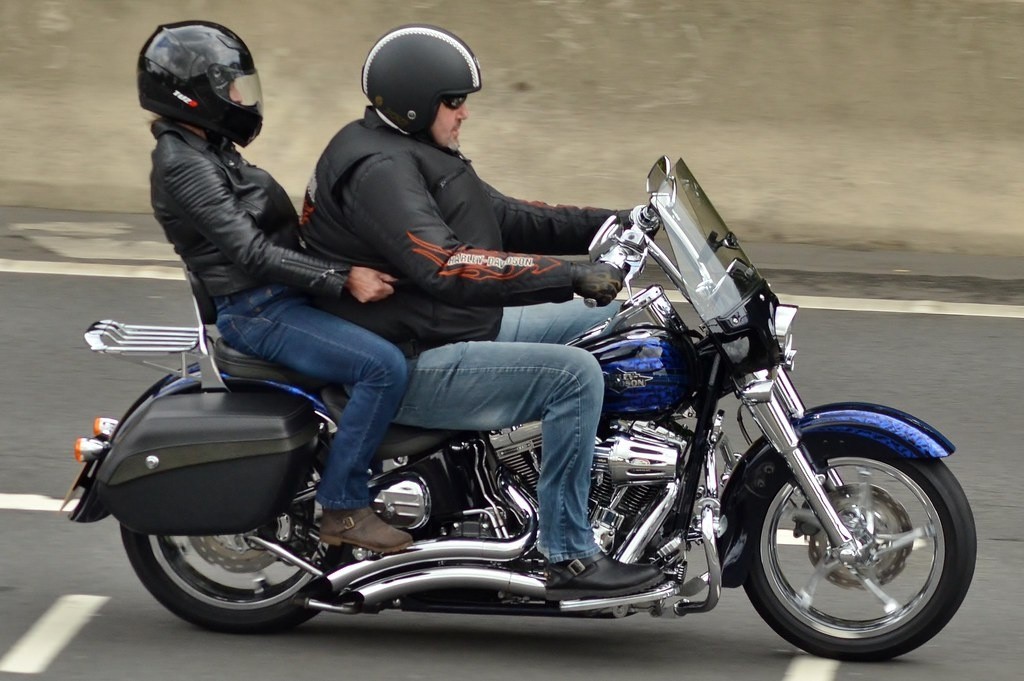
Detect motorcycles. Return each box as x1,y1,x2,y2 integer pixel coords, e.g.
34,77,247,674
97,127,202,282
59,155,977,663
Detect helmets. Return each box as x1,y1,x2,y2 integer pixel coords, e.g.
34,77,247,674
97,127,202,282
361,24,482,134
137,21,263,147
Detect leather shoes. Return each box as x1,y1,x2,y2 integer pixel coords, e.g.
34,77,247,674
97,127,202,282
544,551,664,601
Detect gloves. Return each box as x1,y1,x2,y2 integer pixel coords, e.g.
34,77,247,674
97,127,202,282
572,262,624,306
617,209,632,227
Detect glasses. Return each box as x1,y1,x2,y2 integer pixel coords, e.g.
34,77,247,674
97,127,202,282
442,95,466,109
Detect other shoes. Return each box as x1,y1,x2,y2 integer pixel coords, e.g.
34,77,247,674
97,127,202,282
320,508,414,551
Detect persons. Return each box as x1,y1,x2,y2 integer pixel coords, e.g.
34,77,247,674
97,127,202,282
138,18,414,554
301,25,668,602
